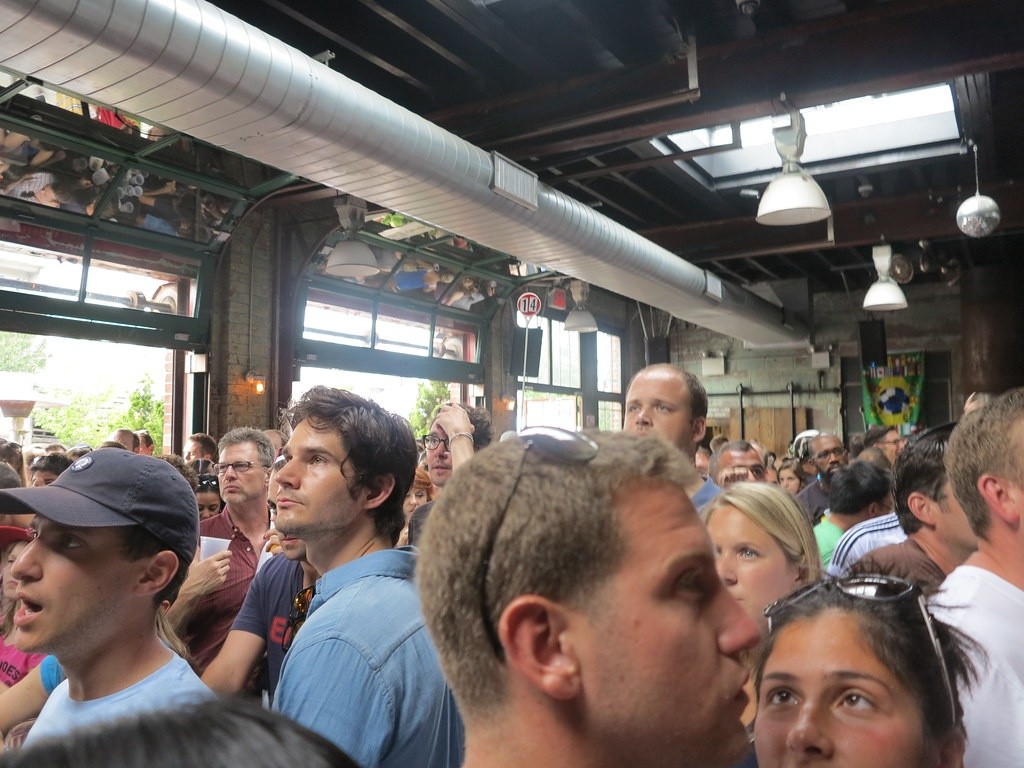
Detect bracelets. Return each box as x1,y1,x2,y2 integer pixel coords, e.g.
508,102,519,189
448,432,474,446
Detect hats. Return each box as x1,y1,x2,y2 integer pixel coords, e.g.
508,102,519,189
0,447,200,562
0,525,34,550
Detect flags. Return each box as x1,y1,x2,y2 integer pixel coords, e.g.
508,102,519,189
862,354,925,439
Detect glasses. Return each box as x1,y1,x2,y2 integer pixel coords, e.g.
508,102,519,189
212,461,271,475
197,478,219,490
281,584,315,652
879,437,906,447
422,434,451,453
478,425,601,663
763,573,958,725
32,455,63,465
811,448,849,462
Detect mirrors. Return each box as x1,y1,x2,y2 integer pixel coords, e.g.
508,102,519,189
475,258,549,278
0,71,22,90
382,256,460,303
426,236,501,263
0,83,172,156
441,272,506,316
144,138,286,191
309,231,406,289
358,211,450,249
0,128,121,217
98,168,235,244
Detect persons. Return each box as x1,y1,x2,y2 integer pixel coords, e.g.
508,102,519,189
339,213,497,313
1,383,465,768
416,359,1024,768
0,75,239,243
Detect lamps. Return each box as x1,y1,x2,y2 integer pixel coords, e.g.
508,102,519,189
245,369,266,394
754,107,831,227
324,196,380,278
562,279,598,334
502,392,516,412
699,349,728,377
862,244,909,312
807,342,835,369
